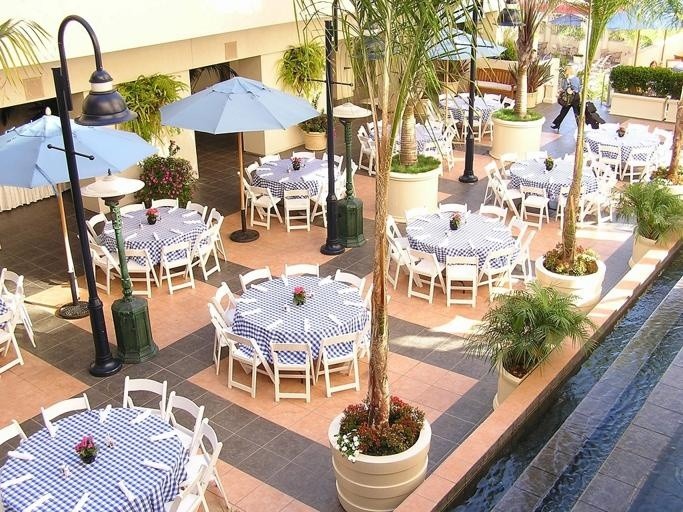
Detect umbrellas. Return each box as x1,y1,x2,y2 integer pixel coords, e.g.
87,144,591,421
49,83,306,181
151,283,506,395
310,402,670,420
425,25,508,126
643,6,683,63
605,9,664,67
0,106,159,306
549,14,588,26
159,76,321,231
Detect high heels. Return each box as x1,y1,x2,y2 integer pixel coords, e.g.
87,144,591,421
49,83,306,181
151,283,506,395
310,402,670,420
549,123,560,131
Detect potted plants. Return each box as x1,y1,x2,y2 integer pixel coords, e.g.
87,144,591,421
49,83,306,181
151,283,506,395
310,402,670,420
458,278,602,413
488,0,683,308
277,0,503,511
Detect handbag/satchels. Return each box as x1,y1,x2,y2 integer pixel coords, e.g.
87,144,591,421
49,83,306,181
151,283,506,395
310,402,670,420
557,90,575,107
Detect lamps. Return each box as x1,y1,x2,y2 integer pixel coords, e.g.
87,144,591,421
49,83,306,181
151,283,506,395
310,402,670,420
50,15,139,376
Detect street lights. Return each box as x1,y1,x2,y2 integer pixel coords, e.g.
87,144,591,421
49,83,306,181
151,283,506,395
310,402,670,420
458,0,524,183
44,15,125,380
319,0,386,255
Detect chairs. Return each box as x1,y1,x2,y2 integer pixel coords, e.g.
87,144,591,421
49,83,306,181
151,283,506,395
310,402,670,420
77,198,226,298
0,376,230,511
0,268,36,373
207,264,316,402
237,153,283,230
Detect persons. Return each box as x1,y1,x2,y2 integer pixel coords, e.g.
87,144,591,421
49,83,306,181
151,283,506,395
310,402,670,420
550,65,582,131
650,61,657,68
